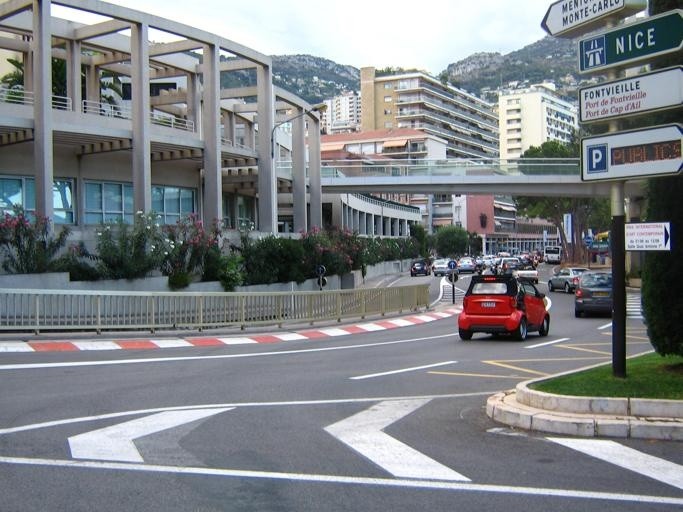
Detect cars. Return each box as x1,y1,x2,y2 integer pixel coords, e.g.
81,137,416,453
458,275,550,340
410,252,541,277
548,268,612,318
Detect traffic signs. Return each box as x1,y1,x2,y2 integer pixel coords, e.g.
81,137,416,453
540,0,647,37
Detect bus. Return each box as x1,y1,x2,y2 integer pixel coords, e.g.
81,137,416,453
543,246,563,263
